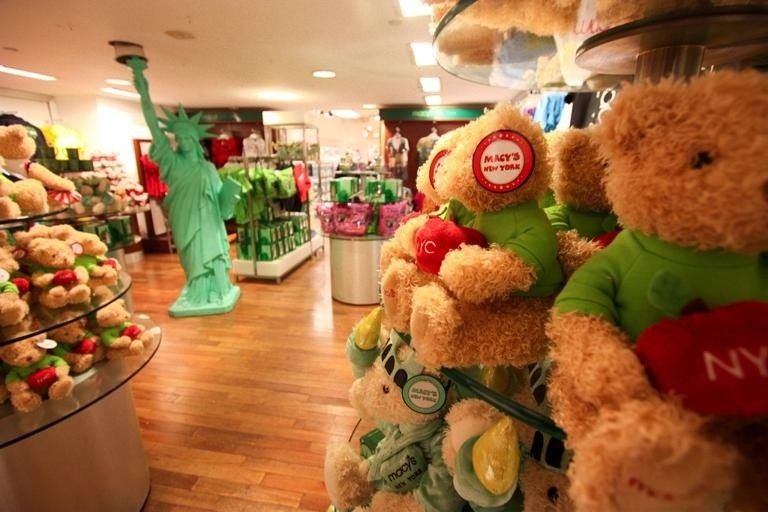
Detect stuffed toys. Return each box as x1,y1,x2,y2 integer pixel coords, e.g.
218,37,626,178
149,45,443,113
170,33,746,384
323,0,768,512
0,123,155,413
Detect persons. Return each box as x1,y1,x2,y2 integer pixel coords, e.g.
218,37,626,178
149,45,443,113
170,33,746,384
127,57,242,317
